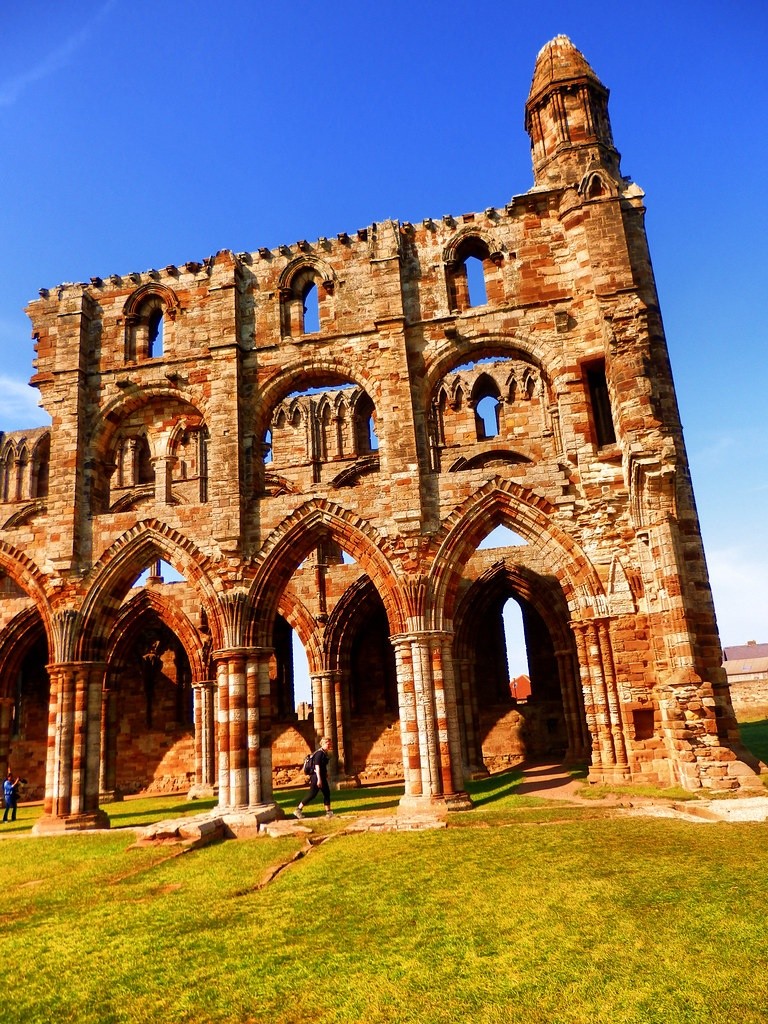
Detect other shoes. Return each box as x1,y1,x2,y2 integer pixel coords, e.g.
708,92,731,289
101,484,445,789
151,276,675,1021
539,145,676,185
293,809,305,819
326,810,336,818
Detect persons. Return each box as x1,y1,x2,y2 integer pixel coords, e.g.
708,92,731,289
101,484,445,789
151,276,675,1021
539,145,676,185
293,737,338,818
3,773,20,823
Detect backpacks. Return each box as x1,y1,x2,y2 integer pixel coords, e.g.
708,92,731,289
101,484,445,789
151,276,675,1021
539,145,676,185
304,750,327,774
